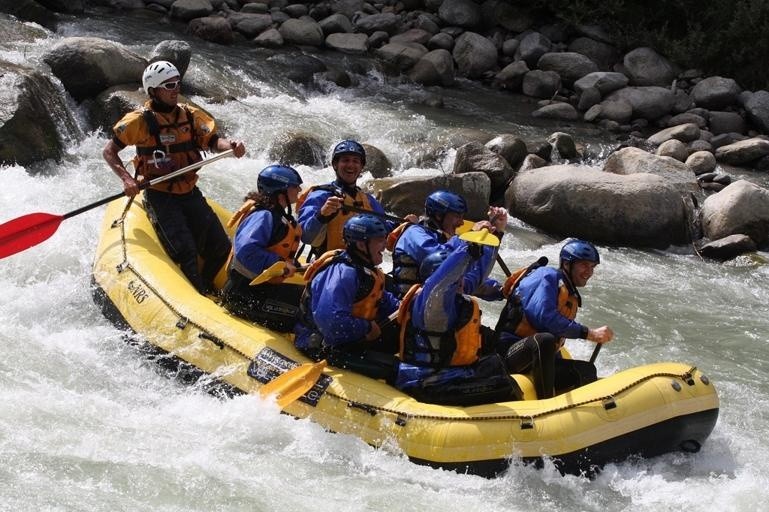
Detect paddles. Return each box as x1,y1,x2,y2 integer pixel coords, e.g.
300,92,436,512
338,202,474,237
0,146,236,260
248,260,311,286
459,207,502,250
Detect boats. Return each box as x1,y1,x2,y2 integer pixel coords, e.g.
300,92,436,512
88,189,720,483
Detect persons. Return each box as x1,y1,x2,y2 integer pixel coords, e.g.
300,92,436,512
397,206,524,407
392,190,512,301
294,213,400,390
220,165,312,334
495,240,613,400
295,141,418,262
103,60,246,297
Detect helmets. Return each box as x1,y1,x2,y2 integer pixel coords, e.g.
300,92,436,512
424,190,467,218
331,140,366,169
343,213,388,245
257,165,303,195
141,61,180,96
419,251,454,280
559,239,600,265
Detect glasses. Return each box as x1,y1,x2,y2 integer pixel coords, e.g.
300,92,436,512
158,79,181,90
